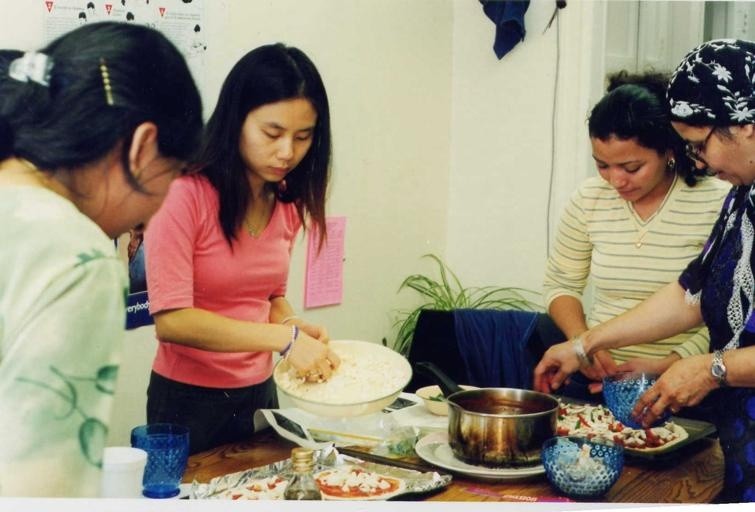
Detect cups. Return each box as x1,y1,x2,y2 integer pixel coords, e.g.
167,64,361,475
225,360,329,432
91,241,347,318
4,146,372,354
129,422,191,498
101,444,148,499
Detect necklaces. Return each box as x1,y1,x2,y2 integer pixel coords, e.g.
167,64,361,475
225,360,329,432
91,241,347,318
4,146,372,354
626,177,678,249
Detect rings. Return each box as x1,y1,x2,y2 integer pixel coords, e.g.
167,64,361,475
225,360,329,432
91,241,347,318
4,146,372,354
307,369,318,377
670,407,678,412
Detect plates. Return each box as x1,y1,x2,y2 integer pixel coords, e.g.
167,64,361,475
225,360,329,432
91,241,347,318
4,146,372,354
414,431,547,482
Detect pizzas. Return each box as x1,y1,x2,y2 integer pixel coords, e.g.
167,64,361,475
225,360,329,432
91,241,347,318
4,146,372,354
223,465,408,500
555,401,689,452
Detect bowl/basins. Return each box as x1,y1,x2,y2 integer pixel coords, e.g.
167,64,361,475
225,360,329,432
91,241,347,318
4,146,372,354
414,383,485,416
540,434,624,499
602,370,673,430
273,339,412,418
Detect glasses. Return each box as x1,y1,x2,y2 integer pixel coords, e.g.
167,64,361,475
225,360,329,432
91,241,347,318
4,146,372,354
683,126,718,162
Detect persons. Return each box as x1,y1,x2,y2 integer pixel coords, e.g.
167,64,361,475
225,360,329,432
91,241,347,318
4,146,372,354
533,37,754,501
1,23,203,499
542,64,733,395
146,42,339,457
127,232,147,292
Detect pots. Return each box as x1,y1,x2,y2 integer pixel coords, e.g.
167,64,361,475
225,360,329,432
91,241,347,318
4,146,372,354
413,358,560,468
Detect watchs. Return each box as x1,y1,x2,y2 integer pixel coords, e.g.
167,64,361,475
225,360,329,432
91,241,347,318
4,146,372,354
711,348,731,389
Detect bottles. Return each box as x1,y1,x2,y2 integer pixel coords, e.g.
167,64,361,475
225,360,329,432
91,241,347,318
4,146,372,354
281,446,323,501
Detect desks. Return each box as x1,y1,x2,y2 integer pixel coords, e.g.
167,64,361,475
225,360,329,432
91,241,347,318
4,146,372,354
180,398,726,503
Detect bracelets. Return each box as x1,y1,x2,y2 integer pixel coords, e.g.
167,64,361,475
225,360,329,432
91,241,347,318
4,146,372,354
573,335,593,368
282,316,300,325
280,325,299,355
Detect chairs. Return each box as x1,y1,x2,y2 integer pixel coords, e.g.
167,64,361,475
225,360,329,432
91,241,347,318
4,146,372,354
409,307,586,389
